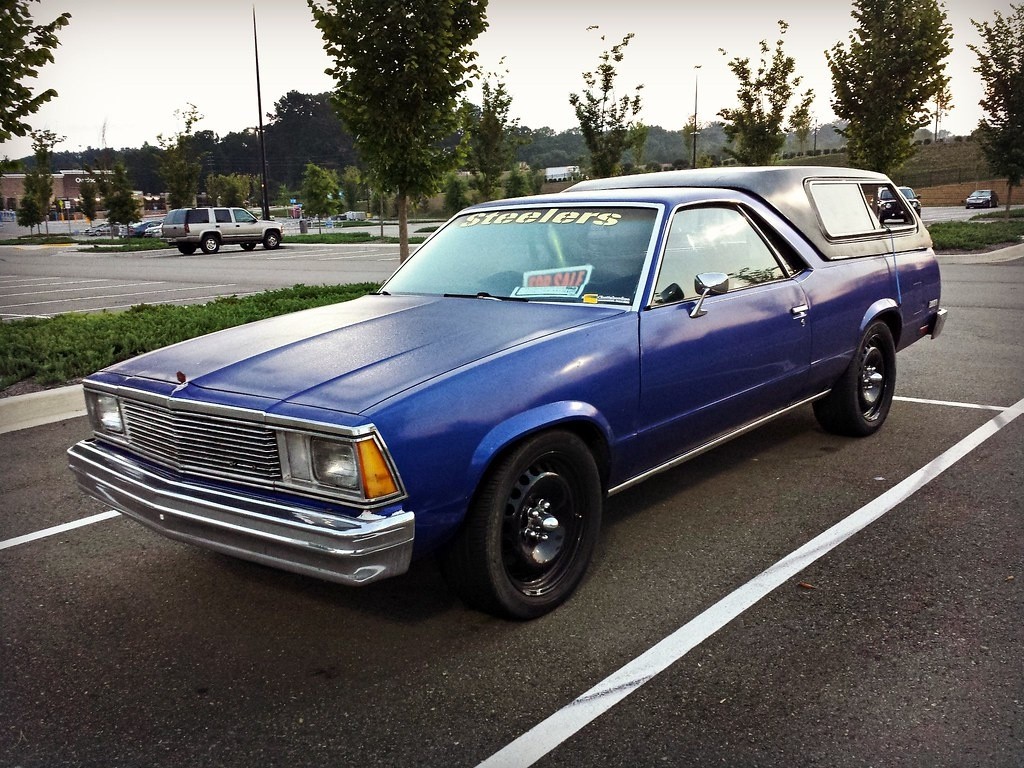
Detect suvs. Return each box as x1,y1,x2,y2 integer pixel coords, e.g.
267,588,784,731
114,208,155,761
162,206,286,255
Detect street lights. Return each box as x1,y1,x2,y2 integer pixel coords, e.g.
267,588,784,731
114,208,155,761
247,126,266,219
691,65,702,168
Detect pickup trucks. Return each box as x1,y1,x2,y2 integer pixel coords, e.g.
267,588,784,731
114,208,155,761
870,186,922,224
66,166,948,623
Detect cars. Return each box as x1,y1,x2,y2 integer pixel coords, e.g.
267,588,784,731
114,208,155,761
88,219,163,239
965,189,999,209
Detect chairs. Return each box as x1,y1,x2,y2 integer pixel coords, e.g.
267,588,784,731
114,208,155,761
325,221,335,233
